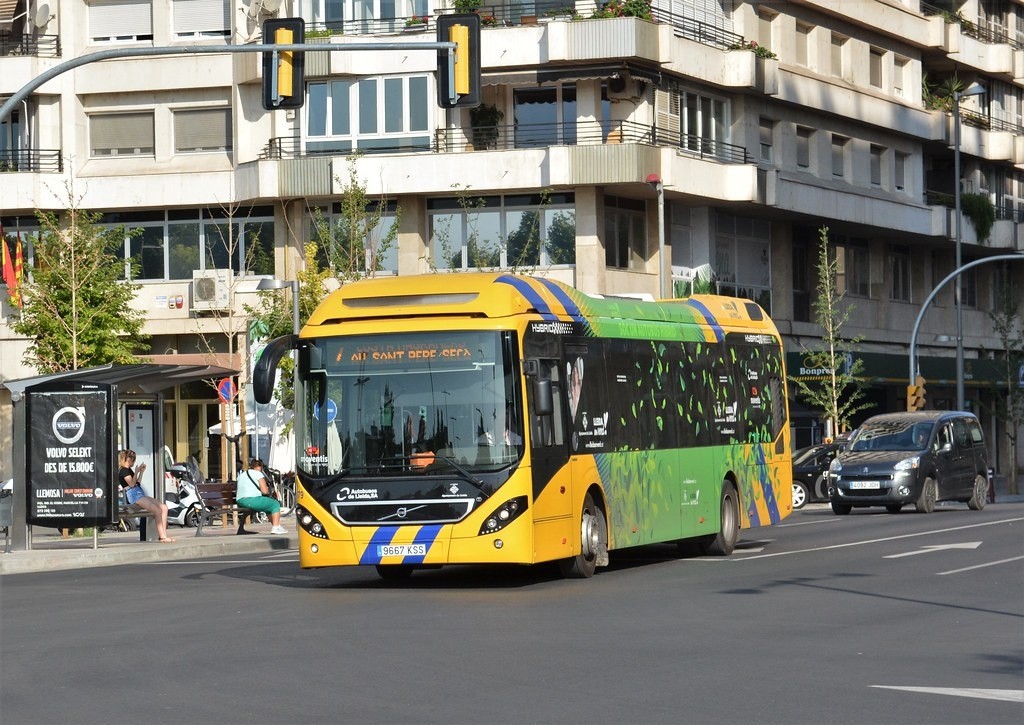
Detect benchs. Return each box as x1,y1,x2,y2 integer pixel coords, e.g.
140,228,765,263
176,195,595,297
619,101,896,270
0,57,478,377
119,510,155,542
195,483,255,540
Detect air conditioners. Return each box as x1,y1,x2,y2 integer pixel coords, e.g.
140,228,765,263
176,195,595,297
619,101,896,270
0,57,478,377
607,77,641,99
193,269,235,309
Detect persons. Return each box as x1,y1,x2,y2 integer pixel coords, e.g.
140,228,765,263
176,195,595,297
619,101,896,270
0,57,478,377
236,459,288,534
917,428,939,451
475,402,521,445
118,450,176,543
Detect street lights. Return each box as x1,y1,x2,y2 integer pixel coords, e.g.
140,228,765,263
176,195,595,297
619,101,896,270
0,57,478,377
255,278,300,372
950,78,987,412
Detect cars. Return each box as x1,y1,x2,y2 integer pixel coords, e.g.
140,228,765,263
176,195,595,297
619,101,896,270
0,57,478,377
790,442,852,509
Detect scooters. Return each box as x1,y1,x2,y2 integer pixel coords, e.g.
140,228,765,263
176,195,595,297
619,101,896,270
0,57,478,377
106,461,213,532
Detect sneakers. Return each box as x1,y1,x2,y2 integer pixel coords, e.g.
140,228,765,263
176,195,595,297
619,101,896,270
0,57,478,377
270,526,288,533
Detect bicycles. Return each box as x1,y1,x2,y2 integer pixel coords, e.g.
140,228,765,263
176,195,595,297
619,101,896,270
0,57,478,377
253,467,297,524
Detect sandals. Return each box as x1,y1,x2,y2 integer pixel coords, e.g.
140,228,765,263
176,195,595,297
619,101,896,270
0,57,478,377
159,537,177,543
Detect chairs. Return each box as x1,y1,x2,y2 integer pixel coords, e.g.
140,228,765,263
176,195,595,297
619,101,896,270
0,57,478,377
503,16,538,26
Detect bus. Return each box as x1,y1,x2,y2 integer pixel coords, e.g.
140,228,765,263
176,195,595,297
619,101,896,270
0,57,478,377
253,273,795,582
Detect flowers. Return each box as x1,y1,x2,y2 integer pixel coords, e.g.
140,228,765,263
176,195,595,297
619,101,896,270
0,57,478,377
405,15,428,24
484,15,496,24
598,0,655,22
744,41,761,51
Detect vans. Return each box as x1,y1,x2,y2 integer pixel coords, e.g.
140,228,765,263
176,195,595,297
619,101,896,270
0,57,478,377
827,410,991,514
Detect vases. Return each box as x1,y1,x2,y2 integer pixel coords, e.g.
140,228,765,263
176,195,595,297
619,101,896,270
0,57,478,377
402,25,426,32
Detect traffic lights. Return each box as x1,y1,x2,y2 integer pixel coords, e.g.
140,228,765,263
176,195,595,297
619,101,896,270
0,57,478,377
906,384,920,411
915,376,928,408
